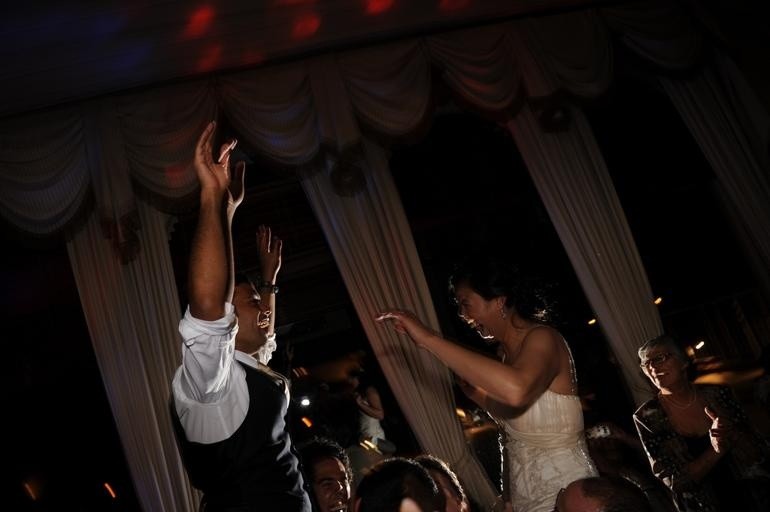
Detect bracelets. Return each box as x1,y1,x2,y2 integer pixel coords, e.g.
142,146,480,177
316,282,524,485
254,274,280,293
659,382,702,411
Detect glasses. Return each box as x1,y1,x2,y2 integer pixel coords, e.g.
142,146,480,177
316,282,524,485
639,351,677,368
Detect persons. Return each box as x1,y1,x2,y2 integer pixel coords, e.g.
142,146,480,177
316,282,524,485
632,333,769,511
168,119,314,512
370,241,602,512
554,476,654,511
352,457,446,511
415,454,467,511
345,365,387,442
294,436,353,512
